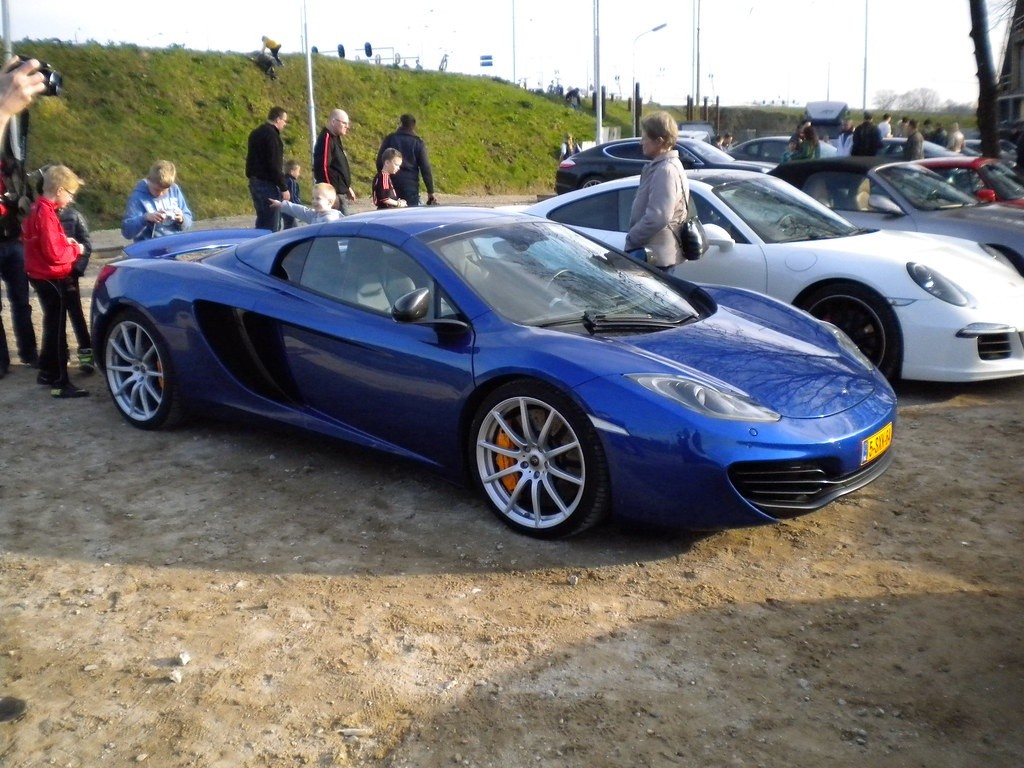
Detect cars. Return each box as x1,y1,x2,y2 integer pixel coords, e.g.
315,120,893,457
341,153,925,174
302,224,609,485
554,135,777,195
878,138,972,179
961,139,1021,157
725,140,839,163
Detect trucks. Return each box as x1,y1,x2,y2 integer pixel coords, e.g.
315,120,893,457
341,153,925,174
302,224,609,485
805,102,850,148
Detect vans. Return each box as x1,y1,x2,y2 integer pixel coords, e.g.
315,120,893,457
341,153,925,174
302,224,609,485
678,121,716,143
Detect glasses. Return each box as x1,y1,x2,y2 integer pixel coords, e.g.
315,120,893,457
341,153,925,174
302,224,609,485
65,189,78,199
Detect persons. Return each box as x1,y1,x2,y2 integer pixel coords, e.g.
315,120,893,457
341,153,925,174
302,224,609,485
0,36,439,397
712,112,1024,164
559,133,583,164
622,110,690,274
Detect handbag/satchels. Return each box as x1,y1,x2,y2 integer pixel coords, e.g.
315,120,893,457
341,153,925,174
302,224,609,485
680,216,709,261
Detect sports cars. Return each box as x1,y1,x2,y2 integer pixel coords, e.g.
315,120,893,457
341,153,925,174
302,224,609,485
736,156,1024,278
907,157,1023,206
470,170,1023,386
90,207,897,542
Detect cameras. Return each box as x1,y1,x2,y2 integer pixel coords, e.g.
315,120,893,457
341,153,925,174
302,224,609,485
8,54,62,96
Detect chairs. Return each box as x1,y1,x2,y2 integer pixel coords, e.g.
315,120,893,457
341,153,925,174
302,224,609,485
808,173,833,208
440,244,490,316
844,175,870,211
337,238,418,313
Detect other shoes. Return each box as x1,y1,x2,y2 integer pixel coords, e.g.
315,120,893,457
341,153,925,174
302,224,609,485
78,354,96,373
51,384,90,398
20,359,39,366
0,367,7,380
38,371,50,384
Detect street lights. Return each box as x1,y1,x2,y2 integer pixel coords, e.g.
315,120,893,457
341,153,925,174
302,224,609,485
632,23,669,138
